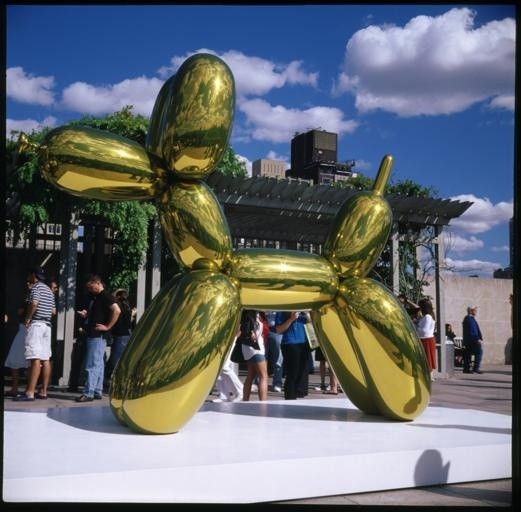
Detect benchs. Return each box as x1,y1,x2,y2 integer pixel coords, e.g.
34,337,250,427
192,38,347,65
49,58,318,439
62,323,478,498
453,338,474,366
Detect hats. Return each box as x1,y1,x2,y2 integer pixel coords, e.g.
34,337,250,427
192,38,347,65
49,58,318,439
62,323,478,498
113,288,126,297
468,304,478,310
31,267,47,281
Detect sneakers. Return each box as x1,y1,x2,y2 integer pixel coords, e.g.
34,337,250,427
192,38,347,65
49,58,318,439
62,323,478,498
93,394,104,400
75,395,93,402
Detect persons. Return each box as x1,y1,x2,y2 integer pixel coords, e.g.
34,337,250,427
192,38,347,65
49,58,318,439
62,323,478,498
461,306,484,374
104,287,132,392
241,309,269,404
314,345,333,391
402,299,436,382
14,271,56,401
211,337,246,404
5,283,57,401
35,281,59,391
323,360,338,395
72,282,114,392
445,325,463,368
398,296,421,320
276,308,312,400
265,310,286,393
76,276,122,402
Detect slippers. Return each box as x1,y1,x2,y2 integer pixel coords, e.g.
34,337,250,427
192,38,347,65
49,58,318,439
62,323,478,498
14,394,34,401
323,390,338,394
35,392,48,400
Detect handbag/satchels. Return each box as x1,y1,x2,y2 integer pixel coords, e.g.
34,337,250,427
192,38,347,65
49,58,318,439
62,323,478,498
303,322,321,349
231,345,248,364
